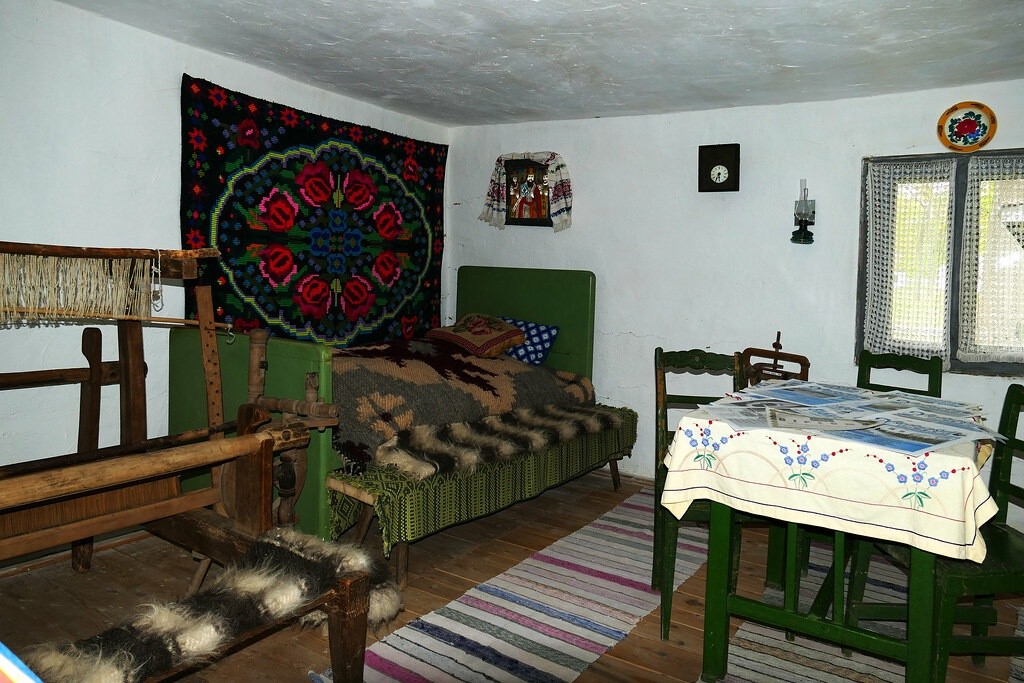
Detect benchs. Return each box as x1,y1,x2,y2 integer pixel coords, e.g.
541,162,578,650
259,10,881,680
21,574,336,683
326,402,637,592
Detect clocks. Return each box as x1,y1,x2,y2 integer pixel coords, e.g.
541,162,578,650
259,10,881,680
698,143,740,192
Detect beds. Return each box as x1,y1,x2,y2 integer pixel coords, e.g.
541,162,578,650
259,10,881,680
168,266,596,544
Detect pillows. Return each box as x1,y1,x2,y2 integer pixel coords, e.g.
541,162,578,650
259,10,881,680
426,312,525,358
497,316,560,367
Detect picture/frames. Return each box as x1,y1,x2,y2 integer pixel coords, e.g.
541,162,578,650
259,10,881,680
504,160,553,227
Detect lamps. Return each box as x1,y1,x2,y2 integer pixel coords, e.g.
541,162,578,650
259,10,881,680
790,177,816,245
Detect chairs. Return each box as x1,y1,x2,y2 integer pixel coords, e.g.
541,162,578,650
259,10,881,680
650,347,804,642
841,385,1024,683
799,348,943,592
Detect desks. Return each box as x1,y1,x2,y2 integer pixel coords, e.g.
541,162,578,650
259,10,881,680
628,379,1000,683
